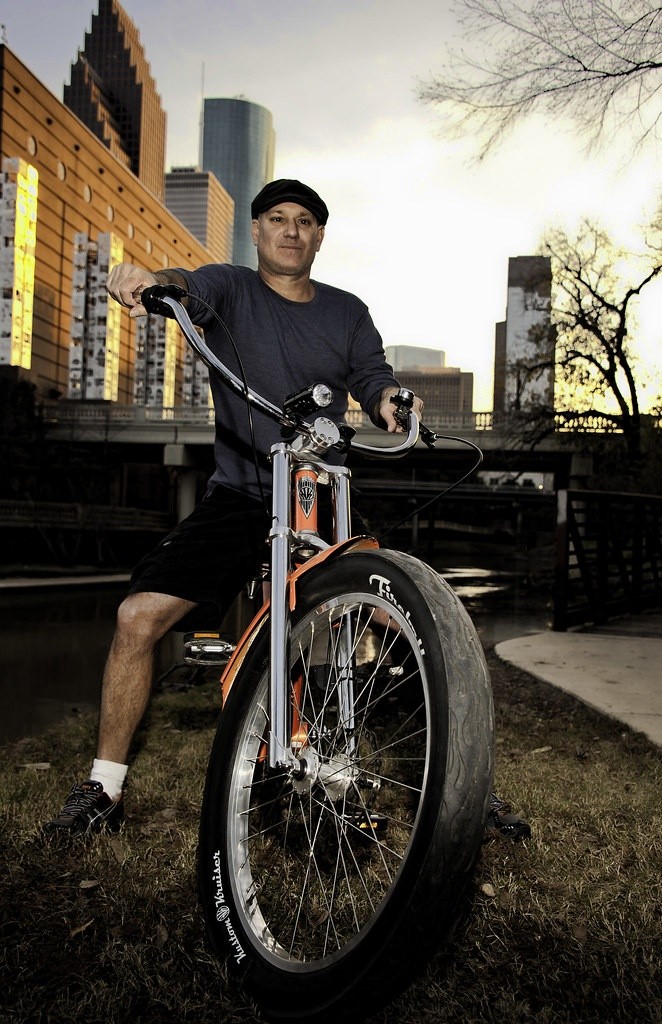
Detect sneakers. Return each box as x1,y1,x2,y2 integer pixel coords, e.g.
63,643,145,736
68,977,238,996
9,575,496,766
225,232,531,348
483,789,531,845
41,780,124,843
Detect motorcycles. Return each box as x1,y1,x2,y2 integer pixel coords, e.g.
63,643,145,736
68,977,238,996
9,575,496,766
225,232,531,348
140,285,498,1023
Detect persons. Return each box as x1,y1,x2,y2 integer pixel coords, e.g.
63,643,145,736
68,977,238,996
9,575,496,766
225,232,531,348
43,178,531,842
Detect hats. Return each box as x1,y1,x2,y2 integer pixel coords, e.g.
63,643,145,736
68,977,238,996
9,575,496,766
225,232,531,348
251,179,329,226
